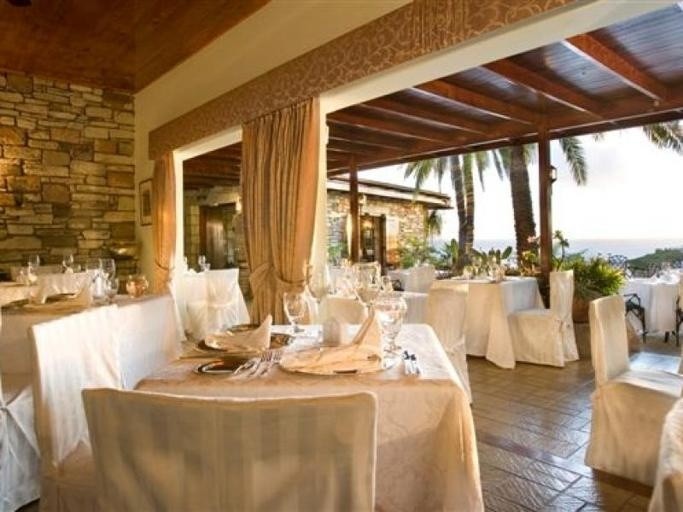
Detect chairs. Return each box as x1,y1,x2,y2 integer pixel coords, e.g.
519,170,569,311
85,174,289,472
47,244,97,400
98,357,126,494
419,287,474,404
81,246,253,336
27,300,119,512
80,384,379,511
585,295,681,485
300,247,682,368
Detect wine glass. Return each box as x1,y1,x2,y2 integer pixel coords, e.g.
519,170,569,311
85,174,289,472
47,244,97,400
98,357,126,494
472,256,497,279
86,258,119,305
282,258,408,353
197,255,206,272
29,253,40,278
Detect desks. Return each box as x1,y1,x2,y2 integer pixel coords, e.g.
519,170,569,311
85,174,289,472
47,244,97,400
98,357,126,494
134,325,484,512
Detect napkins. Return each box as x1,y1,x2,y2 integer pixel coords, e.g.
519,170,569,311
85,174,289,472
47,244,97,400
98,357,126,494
293,311,385,371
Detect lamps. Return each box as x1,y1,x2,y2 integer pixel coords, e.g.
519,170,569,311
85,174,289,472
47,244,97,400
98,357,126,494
360,212,373,231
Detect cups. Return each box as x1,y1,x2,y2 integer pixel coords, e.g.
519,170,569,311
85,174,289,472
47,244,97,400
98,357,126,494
202,262,210,272
126,274,149,301
62,252,75,268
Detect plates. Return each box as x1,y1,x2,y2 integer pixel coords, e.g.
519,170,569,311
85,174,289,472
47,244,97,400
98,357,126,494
196,323,296,375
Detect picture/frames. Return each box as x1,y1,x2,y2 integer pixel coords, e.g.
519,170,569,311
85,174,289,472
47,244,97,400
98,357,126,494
139,179,154,226
